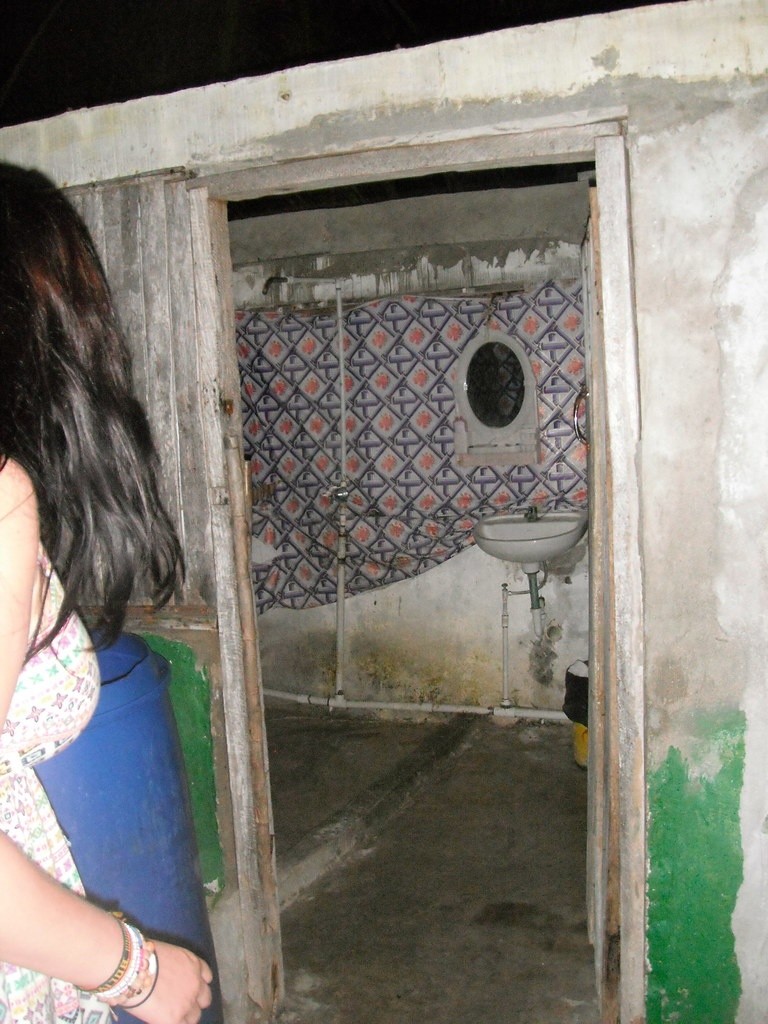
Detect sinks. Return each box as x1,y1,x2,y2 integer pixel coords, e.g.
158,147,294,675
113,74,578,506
472,511,590,563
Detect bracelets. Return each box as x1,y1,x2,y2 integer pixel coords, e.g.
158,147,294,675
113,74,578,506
76,912,159,1013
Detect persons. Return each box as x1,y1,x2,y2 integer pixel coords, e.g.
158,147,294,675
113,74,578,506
0,159,225,1022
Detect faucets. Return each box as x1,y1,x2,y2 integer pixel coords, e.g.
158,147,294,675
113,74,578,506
524,506,539,523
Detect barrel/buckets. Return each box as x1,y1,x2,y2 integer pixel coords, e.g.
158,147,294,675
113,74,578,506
34,630,223,1024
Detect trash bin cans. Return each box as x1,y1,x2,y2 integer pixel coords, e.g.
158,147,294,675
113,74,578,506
564,659,588,771
